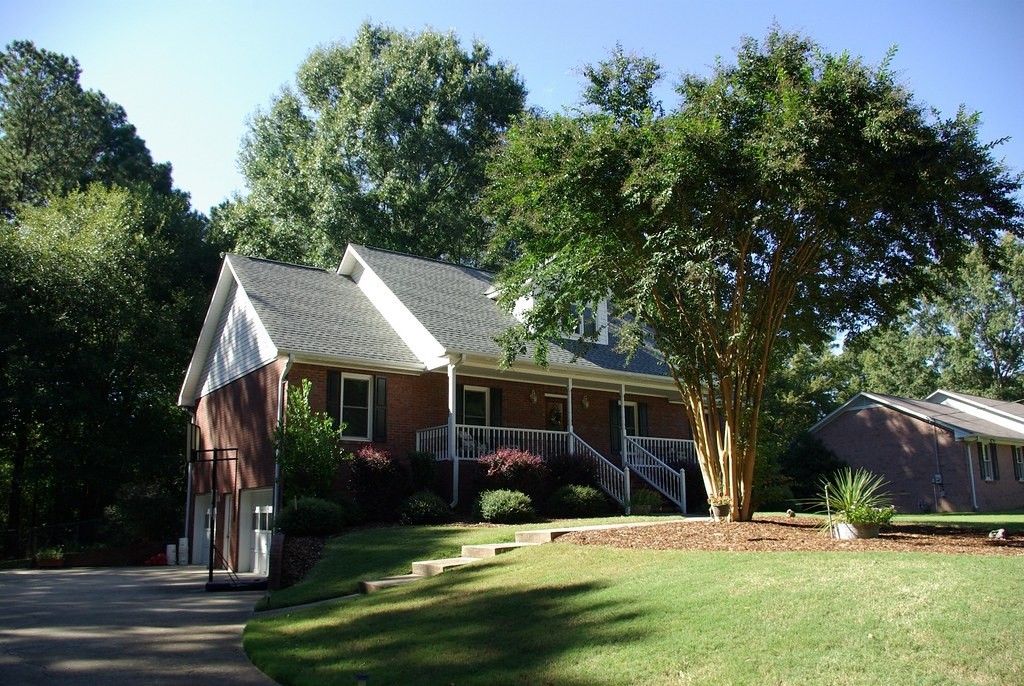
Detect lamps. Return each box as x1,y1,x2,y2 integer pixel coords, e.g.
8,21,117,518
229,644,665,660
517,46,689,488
529,389,538,405
582,395,589,409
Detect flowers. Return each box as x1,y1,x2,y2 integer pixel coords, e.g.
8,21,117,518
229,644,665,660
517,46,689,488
785,466,897,535
707,493,732,506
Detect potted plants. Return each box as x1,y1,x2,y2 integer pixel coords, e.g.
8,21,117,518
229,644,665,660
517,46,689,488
560,484,608,514
33,545,65,568
631,489,665,515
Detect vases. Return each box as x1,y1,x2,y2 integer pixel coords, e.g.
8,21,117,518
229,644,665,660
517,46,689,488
713,505,730,516
833,523,879,539
